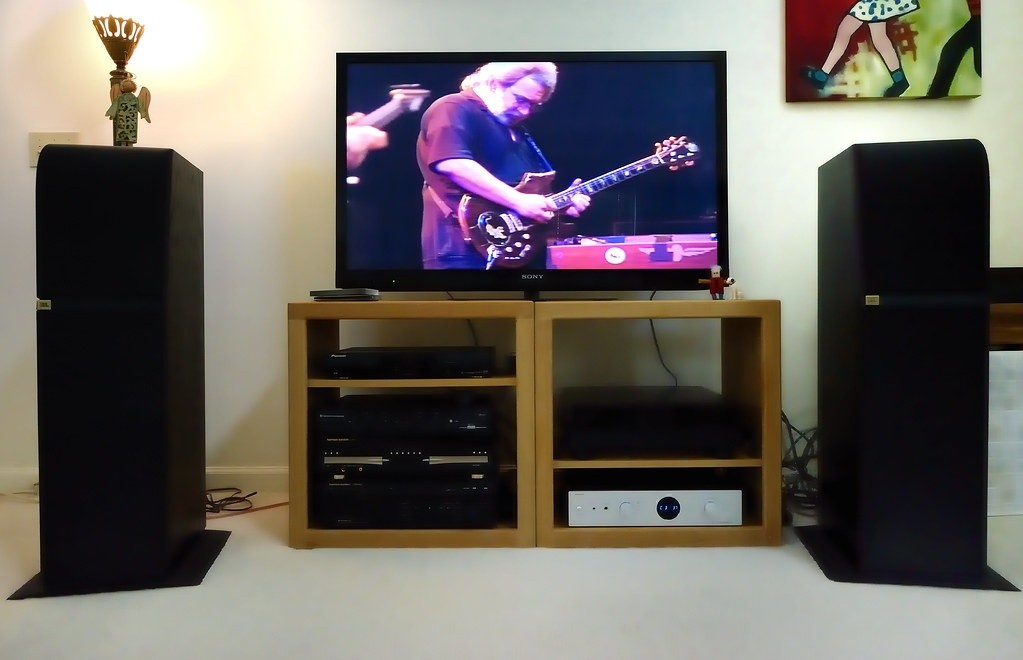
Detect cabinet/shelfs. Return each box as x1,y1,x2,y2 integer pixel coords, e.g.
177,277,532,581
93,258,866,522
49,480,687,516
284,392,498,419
284,288,785,549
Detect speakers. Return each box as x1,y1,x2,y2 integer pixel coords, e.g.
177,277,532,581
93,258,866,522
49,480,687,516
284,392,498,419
32,143,205,587
814,139,991,576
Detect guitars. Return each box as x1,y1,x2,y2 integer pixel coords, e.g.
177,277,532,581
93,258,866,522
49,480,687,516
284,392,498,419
456,137,699,269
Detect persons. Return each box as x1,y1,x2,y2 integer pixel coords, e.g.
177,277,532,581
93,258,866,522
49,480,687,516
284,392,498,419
345,110,389,173
416,61,590,271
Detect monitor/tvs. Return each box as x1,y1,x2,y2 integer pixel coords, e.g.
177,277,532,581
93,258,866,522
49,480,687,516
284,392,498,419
335,51,730,289
